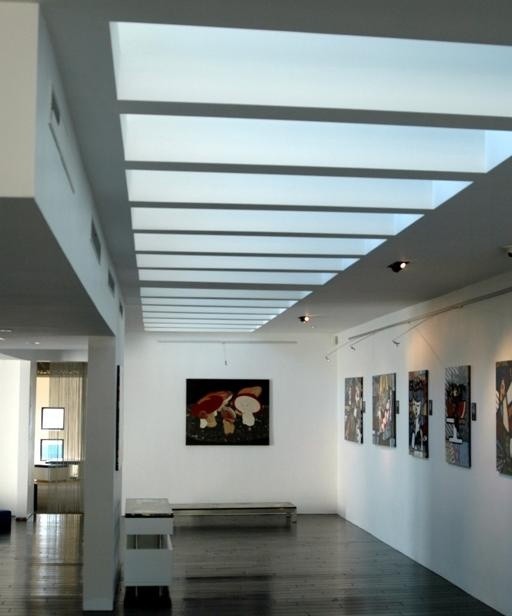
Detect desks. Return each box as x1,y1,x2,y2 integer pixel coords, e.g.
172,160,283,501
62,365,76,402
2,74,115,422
122,496,176,615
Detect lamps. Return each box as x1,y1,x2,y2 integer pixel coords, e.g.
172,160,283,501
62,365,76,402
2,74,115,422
388,259,409,273
298,316,310,322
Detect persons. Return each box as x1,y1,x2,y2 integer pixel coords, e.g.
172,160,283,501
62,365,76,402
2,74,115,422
409,378,427,452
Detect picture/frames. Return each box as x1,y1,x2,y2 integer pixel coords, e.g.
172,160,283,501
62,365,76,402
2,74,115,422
40,406,65,462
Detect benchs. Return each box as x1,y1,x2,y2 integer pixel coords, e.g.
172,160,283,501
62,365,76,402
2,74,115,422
171,501,298,528
34,464,72,483
